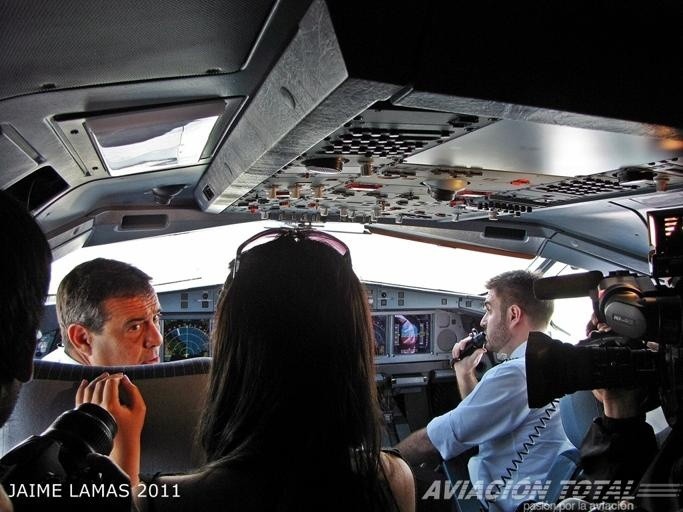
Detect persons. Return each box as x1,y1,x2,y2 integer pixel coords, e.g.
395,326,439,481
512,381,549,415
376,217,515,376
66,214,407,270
0,185,51,511
71,225,417,510
37,254,163,367
389,264,604,511
555,320,682,511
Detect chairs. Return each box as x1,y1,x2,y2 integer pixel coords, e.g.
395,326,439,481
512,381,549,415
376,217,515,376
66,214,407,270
0,355,221,511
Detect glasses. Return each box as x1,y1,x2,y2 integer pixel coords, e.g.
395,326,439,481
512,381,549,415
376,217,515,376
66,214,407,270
232,229,350,279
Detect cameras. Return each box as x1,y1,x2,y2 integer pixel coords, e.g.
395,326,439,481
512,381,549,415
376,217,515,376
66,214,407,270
0,402,118,512
